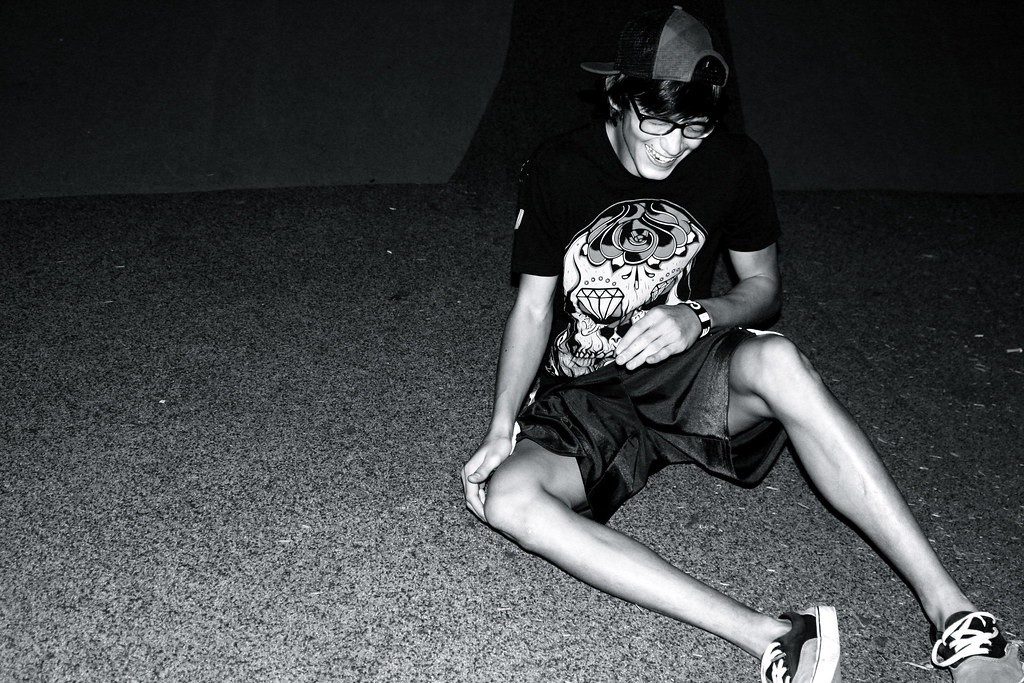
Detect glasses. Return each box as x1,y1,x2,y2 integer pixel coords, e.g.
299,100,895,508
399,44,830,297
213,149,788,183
626,91,717,139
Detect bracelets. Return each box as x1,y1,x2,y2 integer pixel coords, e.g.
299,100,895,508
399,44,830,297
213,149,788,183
677,301,711,338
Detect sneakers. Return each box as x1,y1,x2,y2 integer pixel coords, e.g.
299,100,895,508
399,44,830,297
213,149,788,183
931,610,1024,683
760,606,841,683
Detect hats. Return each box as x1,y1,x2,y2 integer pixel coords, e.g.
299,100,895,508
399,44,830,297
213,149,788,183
579,4,729,89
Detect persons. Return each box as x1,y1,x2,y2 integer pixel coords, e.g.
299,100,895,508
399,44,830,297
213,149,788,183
461,4,1024,683
